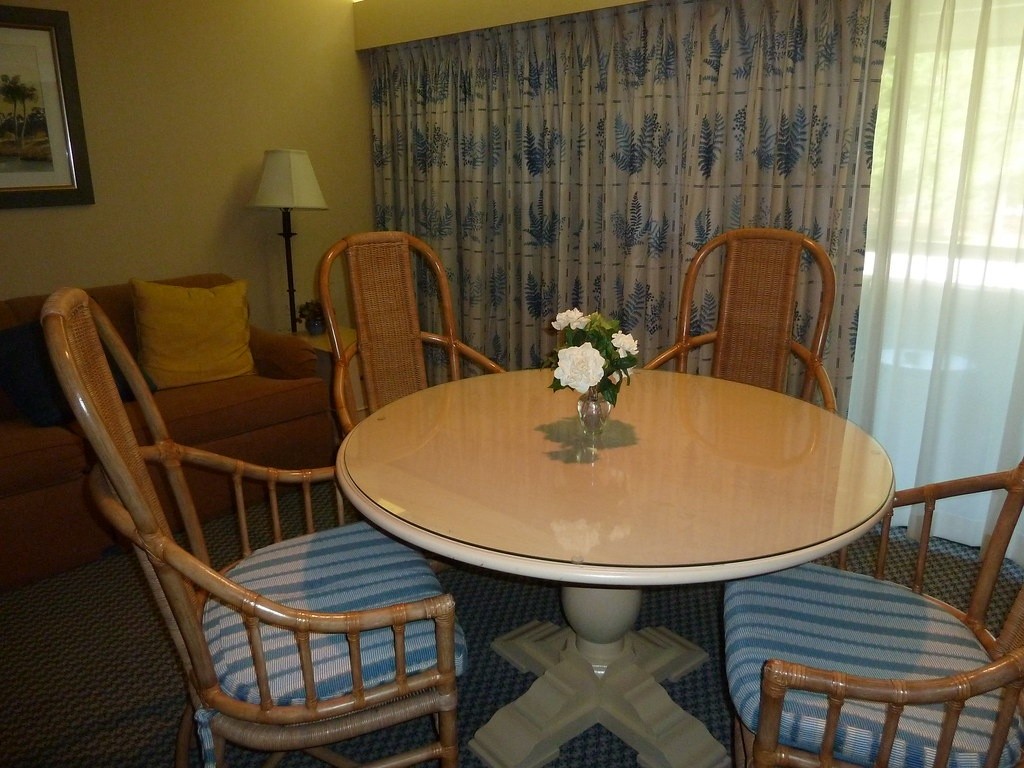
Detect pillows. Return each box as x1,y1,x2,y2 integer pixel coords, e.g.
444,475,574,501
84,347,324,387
127,278,255,391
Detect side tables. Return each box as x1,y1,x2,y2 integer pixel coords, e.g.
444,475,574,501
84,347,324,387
279,322,358,369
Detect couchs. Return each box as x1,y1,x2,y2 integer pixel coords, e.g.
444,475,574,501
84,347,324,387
0,272,333,586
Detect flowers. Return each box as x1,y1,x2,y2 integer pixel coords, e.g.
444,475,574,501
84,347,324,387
548,307,641,395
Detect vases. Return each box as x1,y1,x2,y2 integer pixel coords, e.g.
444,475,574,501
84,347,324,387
577,433,607,479
577,387,614,433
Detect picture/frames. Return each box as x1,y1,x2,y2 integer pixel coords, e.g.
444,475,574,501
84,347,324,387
0,4,97,209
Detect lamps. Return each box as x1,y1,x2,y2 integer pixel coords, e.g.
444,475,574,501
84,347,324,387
244,150,329,340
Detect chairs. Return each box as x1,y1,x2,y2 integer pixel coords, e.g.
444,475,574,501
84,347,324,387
315,229,511,434
39,285,467,765
634,229,840,415
726,447,1023,768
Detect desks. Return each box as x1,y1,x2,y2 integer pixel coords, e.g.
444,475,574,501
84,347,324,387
333,368,897,768
